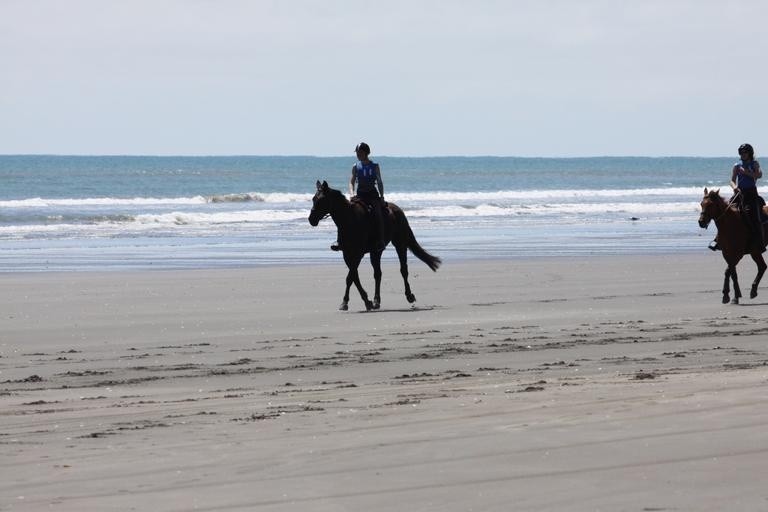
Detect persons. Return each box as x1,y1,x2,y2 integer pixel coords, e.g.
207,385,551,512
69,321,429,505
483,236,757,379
707,143,768,251
328,143,385,249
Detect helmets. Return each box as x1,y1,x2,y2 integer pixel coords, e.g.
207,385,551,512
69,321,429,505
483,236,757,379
355,143,370,154
738,143,753,155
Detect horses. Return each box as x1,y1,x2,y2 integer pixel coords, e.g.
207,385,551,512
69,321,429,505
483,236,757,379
697,187,768,304
307,179,442,311
